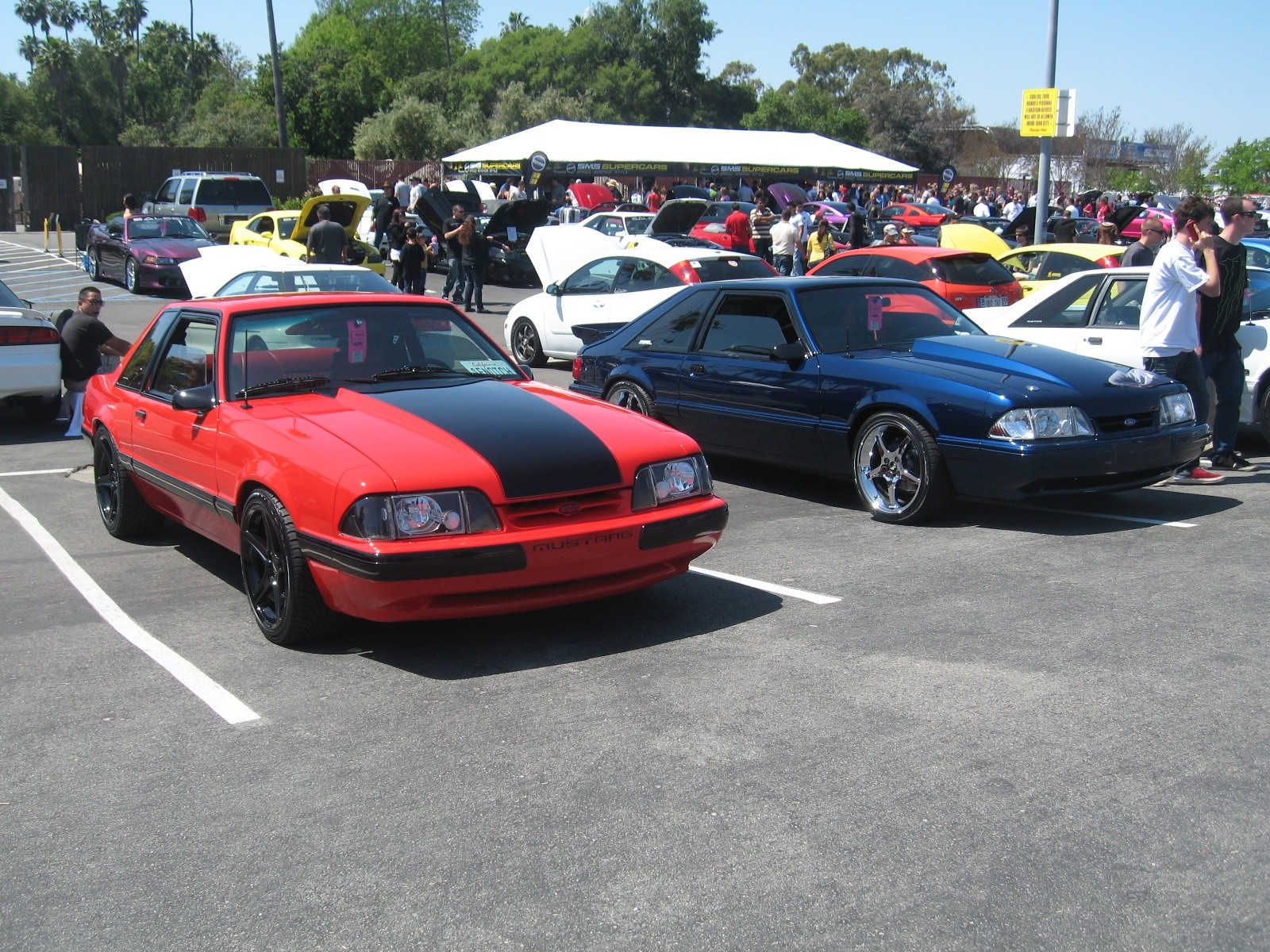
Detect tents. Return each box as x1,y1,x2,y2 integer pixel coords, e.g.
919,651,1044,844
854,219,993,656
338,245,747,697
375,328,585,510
439,119,920,203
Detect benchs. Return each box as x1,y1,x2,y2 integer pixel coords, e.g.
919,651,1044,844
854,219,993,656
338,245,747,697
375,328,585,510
161,345,337,396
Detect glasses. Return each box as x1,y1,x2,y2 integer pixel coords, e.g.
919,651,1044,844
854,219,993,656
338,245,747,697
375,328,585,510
455,210,464,213
1015,234,1024,237
1146,229,1163,235
81,298,105,307
1226,211,1257,218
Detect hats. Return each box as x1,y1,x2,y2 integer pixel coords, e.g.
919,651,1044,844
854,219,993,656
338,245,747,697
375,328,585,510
902,228,915,234
883,223,898,236
409,176,418,181
382,180,393,189
815,210,823,217
841,187,848,193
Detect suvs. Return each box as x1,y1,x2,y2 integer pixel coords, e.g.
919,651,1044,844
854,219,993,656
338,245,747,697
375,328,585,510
141,171,277,233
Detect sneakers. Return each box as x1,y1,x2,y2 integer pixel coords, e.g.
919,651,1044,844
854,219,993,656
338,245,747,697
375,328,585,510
1210,451,1261,473
1170,466,1225,486
1150,479,1169,488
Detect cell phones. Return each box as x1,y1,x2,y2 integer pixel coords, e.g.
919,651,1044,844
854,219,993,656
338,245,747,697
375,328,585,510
1185,220,1200,242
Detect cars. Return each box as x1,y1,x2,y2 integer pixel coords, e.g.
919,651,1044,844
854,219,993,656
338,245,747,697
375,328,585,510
318,180,1270,343
805,246,1025,328
81,291,730,648
568,273,1214,528
936,223,1129,307
952,263,1270,455
0,280,63,422
185,244,456,354
504,223,783,369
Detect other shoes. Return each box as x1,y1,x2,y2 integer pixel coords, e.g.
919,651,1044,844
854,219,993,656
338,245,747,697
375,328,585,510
453,297,465,304
464,307,476,312
442,295,448,301
476,309,492,314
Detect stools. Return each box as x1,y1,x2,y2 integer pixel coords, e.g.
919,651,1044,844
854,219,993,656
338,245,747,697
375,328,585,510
62,390,84,436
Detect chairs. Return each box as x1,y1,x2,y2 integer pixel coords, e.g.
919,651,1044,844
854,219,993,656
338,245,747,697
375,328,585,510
635,223,648,235
1106,305,1139,325
1252,251,1269,270
628,269,656,290
74,223,90,270
160,219,182,238
608,221,619,237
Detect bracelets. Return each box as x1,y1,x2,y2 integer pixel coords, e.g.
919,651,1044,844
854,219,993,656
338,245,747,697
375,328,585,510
305,255,310,258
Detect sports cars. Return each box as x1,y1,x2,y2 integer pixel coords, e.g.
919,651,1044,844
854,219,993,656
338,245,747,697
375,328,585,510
228,193,386,279
85,211,217,294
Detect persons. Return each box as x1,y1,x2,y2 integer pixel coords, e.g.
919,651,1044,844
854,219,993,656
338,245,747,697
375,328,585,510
1193,197,1258,471
124,193,141,218
490,178,527,200
429,235,439,254
725,198,835,276
305,206,350,264
704,182,762,204
551,178,582,202
1052,192,1140,245
1117,217,1163,295
60,287,132,392
808,183,1037,222
1016,224,1031,248
601,179,667,212
394,174,511,313
1139,196,1221,486
880,224,915,245
332,185,340,194
845,202,863,250
371,181,400,250
